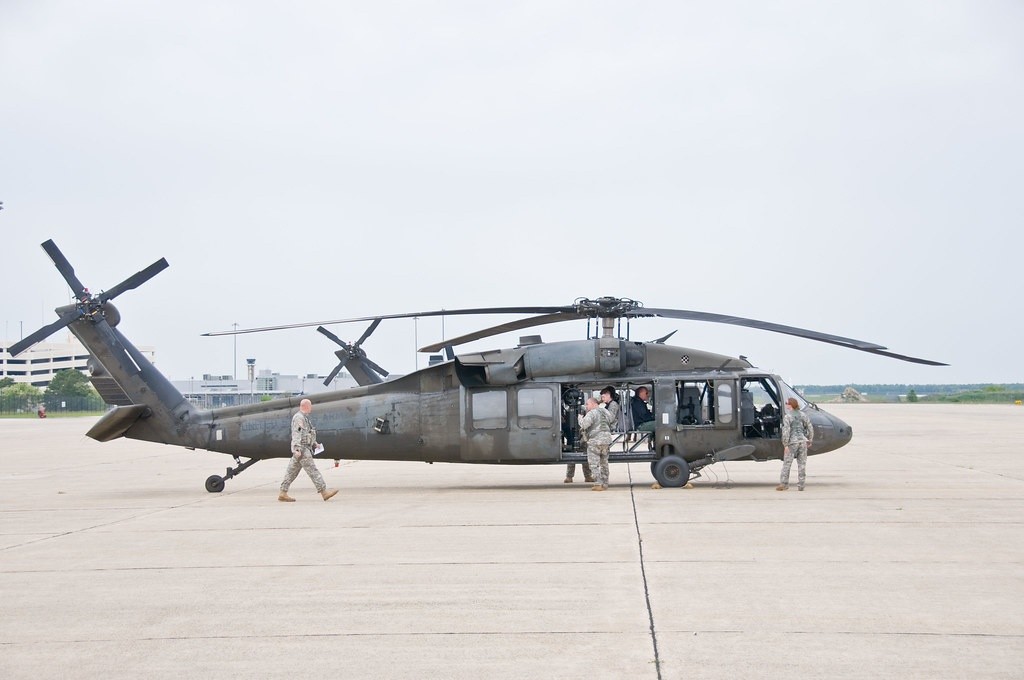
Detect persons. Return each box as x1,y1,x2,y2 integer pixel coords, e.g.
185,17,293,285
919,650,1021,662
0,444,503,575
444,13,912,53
776,397,814,491
560,463,594,482
594,386,622,432
631,386,654,431
577,399,614,491
278,399,339,503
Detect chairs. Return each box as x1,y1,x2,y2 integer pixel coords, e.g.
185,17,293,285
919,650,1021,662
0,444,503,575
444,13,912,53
607,389,626,453
624,390,654,452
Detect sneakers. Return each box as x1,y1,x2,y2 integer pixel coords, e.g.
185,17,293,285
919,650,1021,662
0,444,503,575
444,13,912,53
775,486,784,491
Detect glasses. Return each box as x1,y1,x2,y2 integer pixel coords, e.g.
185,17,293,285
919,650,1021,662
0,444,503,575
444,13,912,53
785,402,788,405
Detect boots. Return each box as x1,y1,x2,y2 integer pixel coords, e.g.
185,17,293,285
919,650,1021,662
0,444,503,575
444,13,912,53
592,485,604,491
320,489,339,501
278,490,296,502
564,477,573,483
585,477,595,482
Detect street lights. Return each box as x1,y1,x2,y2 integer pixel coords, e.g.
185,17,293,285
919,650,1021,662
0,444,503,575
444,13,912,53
231,323,240,380
412,317,419,372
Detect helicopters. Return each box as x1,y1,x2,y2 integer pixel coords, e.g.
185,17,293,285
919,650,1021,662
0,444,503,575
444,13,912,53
7,236,951,494
